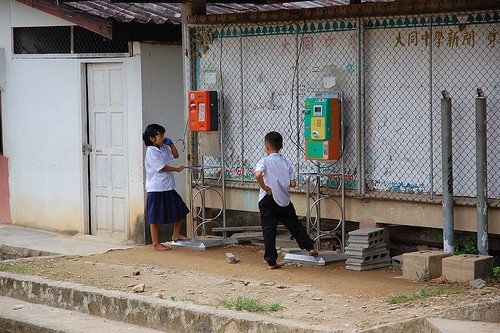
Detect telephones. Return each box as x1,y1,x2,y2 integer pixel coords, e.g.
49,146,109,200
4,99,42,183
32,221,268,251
164,138,171,145
303,97,339,161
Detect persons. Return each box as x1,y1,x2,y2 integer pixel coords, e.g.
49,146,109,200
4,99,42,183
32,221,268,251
255,131,318,269
142,124,192,251
